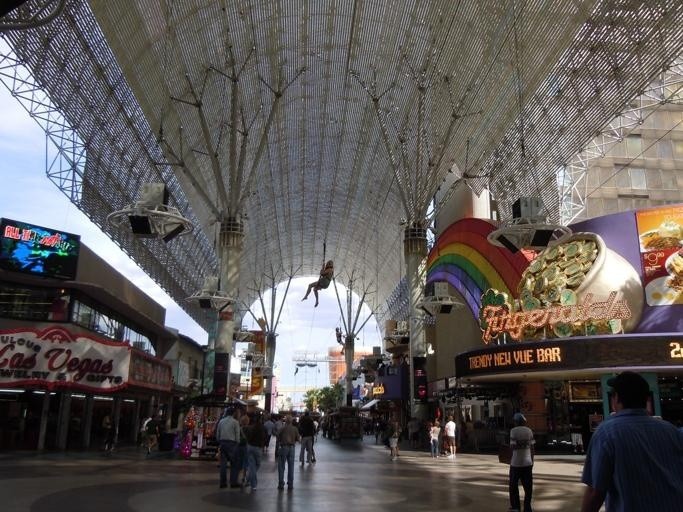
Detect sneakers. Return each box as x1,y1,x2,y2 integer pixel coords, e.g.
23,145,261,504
218,477,294,490
508,508,521,511
429,452,456,459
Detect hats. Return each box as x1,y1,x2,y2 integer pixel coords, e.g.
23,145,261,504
607,372,649,399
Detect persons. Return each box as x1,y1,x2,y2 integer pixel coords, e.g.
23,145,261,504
140,414,160,456
301,260,334,307
336,327,346,355
364,416,457,460
102,415,117,452
216,407,319,491
580,371,683,512
508,413,536,512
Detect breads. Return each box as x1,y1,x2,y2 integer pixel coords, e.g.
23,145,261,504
658,221,683,240
